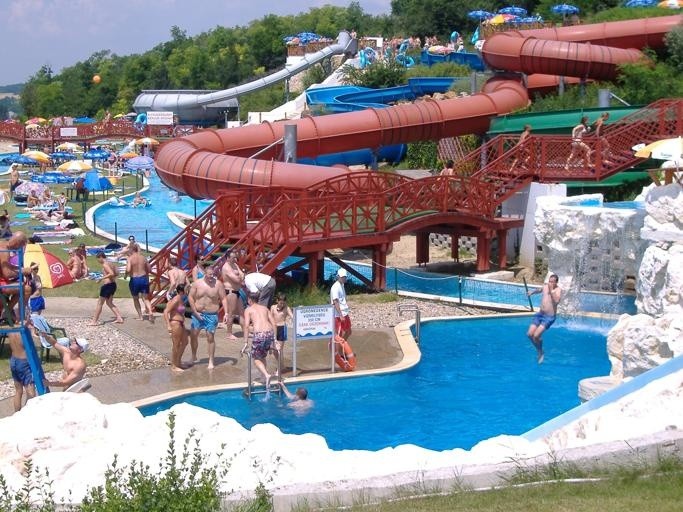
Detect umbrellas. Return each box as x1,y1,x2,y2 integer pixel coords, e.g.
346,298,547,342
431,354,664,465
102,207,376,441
282,31,332,47
632,135,683,188
463,1,682,37
0,109,160,186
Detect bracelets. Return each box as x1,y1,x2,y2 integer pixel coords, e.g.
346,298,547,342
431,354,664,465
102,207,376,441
550,290,554,294
243,341,247,343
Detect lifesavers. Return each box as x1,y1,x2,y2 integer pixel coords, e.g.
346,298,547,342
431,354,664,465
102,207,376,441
131,197,151,209
328,336,356,371
109,202,127,207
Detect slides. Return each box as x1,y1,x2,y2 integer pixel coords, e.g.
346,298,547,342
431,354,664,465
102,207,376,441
134,29,359,126
151,14,683,200
133,16,682,200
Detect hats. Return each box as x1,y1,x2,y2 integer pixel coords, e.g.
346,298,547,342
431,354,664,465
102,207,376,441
338,268,347,278
73,336,90,353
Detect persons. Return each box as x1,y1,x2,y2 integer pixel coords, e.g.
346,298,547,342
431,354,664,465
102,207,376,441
331,25,465,74
38,328,91,393
0,163,89,244
589,110,613,168
238,292,281,391
6,304,43,414
277,378,315,418
172,192,181,204
430,160,455,194
111,235,140,263
29,262,44,317
63,241,90,281
525,274,562,366
329,267,351,359
0,231,32,326
508,125,533,175
108,192,149,208
87,244,293,377
563,115,590,171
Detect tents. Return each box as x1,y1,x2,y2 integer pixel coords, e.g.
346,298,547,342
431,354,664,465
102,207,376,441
22,241,74,289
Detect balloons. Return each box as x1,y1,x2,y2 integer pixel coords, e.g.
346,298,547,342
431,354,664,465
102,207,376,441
92,75,101,84
